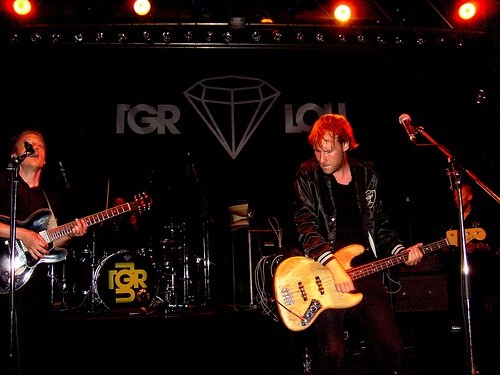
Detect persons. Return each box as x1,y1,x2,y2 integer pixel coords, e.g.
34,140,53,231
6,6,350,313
100,198,137,231
451,184,500,330
0,129,87,375
295,113,423,375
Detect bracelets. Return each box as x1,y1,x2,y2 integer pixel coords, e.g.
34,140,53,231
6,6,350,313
68,233,72,238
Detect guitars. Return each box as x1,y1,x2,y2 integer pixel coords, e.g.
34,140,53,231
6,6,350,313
272,220,487,336
0,192,154,294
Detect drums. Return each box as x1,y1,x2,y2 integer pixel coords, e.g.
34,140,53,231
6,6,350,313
159,222,187,246
24,249,68,309
93,251,159,313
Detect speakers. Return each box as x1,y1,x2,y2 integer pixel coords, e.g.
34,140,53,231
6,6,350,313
392,250,450,315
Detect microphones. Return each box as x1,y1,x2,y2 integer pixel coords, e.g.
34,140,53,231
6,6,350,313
23,141,37,157
399,114,417,145
185,152,190,177
58,162,70,188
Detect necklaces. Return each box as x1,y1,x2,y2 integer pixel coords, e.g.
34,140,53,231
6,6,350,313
113,214,121,231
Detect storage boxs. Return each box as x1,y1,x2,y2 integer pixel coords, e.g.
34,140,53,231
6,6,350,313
231,229,284,306
